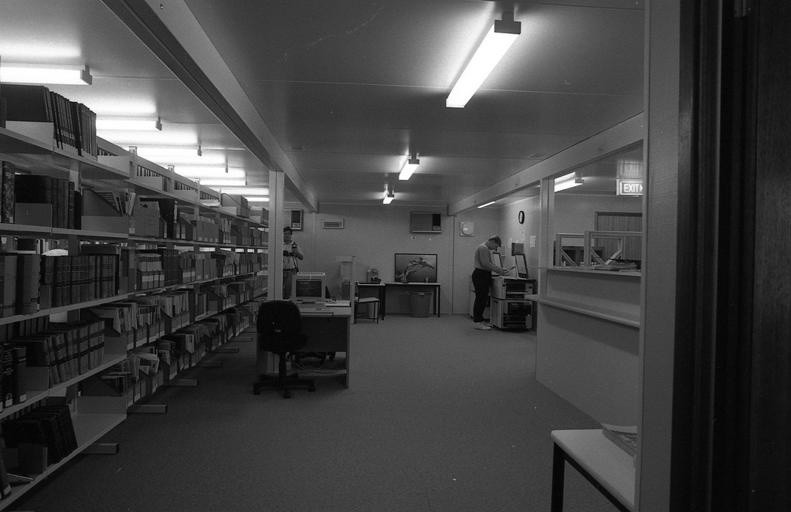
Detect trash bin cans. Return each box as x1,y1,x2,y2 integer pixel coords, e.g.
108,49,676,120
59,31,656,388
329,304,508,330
408,290,432,316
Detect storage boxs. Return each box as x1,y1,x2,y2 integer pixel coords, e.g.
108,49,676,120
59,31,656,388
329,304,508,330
249,206,270,224
221,193,248,218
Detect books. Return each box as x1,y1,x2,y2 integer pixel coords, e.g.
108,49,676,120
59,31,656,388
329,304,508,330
1,84,269,499
325,299,351,307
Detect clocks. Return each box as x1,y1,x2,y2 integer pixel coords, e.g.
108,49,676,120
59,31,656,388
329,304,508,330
518,211,526,223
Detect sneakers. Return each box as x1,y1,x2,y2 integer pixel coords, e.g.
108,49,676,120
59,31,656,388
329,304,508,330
472,321,491,330
482,320,491,326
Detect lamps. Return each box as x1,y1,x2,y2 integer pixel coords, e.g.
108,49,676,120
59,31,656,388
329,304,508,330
444,5,529,109
398,148,421,182
554,178,586,193
382,184,396,206
0,60,253,187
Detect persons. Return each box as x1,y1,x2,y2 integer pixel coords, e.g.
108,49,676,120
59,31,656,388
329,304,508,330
471,235,501,330
281,226,304,299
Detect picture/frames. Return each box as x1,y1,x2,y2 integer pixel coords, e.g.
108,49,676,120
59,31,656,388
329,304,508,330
394,253,438,284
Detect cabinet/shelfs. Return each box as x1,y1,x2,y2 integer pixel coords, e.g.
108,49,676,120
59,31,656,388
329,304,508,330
243,214,269,333
199,189,242,367
132,154,198,414
0,131,133,511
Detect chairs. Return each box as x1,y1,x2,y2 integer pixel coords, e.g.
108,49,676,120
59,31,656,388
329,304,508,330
348,280,380,323
254,299,311,397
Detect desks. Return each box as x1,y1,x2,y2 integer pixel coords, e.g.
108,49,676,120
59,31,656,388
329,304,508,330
257,296,353,388
383,283,443,320
550,425,639,511
256,271,328,298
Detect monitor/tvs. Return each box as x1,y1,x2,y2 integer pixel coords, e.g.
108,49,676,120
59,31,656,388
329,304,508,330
493,252,503,267
515,253,528,278
